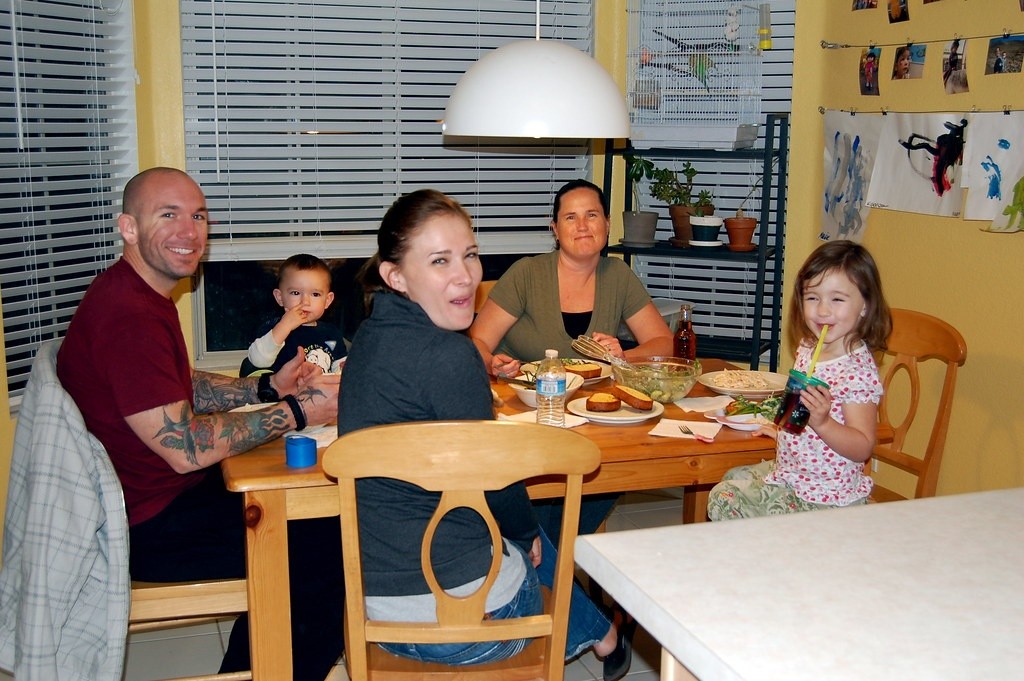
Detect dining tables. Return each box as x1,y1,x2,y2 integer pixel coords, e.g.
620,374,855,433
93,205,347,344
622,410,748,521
220,358,776,681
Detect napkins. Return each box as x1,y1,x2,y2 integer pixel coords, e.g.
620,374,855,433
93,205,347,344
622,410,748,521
497,410,590,429
648,417,724,440
672,395,736,413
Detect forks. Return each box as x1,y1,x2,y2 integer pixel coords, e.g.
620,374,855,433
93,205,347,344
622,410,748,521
678,425,714,443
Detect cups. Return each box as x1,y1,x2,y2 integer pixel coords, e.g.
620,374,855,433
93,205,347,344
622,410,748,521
285,433,317,469
775,371,831,436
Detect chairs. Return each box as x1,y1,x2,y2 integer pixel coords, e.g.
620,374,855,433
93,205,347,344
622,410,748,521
322,420,602,681
865,308,968,503
32,338,252,681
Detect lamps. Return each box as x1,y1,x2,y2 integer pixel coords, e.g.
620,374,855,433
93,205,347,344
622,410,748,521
441,0,632,139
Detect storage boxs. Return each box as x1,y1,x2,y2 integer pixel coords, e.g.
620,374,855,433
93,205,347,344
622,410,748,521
617,297,697,341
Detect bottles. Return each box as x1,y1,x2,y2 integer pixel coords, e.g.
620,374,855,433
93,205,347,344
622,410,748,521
536,348,566,429
671,304,696,372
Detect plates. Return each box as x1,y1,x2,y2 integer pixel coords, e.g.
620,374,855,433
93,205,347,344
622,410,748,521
567,395,665,424
698,370,790,399
229,402,322,437
519,358,614,386
702,408,765,432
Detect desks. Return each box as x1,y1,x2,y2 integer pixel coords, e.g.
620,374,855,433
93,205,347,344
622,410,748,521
573,486,1024,681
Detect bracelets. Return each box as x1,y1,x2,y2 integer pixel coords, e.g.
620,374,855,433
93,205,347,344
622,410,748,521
257,372,277,403
284,394,307,431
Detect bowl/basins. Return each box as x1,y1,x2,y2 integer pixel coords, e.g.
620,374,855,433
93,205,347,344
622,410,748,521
508,372,585,408
611,354,703,404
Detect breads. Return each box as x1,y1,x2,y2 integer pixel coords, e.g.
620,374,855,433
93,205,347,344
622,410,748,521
563,363,602,378
585,393,621,411
612,384,653,410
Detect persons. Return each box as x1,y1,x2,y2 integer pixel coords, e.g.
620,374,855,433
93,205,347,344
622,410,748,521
240,254,349,377
943,40,960,88
336,189,632,681
706,241,894,522
53,167,344,681
892,48,910,79
467,180,675,554
864,53,875,90
994,47,1006,73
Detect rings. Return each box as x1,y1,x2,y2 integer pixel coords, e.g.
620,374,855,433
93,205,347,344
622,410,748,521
607,345,611,351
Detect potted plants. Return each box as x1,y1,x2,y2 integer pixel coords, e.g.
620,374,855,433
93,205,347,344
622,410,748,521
619,155,779,250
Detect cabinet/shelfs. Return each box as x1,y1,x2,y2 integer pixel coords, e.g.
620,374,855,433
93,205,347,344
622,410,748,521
600,111,789,374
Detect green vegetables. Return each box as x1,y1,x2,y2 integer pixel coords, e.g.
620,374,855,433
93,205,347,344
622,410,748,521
617,358,700,400
726,393,781,420
560,358,574,364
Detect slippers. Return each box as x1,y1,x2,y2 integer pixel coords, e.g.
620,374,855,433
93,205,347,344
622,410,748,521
602,604,636,681
